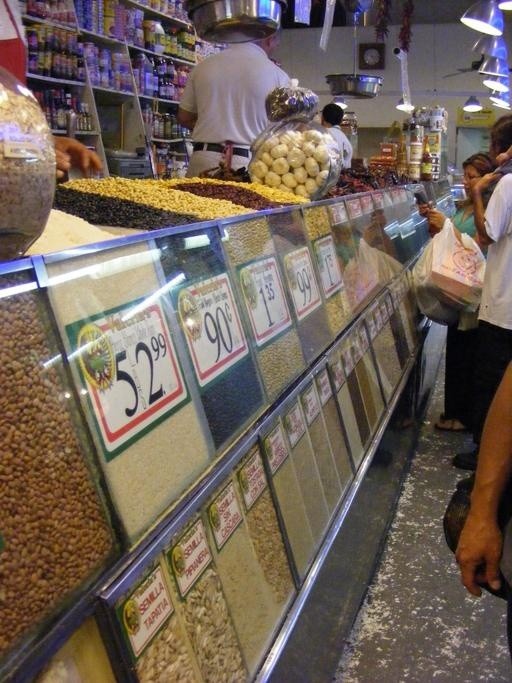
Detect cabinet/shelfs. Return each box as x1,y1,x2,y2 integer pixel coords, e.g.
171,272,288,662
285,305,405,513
1,175,452,683
0,0,231,178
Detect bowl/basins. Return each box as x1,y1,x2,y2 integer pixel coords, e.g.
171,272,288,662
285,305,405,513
326,74,384,99
187,1,288,44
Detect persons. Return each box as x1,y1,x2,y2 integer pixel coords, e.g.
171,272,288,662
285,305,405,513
177,30,291,178
418,153,494,427
322,103,353,169
453,115,512,471
0,0,104,178
495,146,512,166
455,359,512,664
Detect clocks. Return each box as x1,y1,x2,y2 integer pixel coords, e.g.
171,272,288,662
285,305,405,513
359,43,384,69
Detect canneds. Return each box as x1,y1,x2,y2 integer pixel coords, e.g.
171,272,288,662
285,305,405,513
410,142,422,162
408,162,421,183
167,26,195,62
26,23,85,83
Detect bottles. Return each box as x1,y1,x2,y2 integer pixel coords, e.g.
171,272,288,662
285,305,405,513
421,135,432,182
155,18,166,53
134,53,192,101
35,88,92,131
143,106,190,140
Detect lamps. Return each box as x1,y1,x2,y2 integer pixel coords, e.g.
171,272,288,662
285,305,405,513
472,32,509,61
499,0,511,12
484,75,510,94
477,58,510,77
491,104,511,112
489,95,510,105
329,98,347,110
463,96,483,112
460,1,505,38
395,97,415,112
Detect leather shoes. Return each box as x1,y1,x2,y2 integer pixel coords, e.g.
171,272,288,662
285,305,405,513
452,447,480,470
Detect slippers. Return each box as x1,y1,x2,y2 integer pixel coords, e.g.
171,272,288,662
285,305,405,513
435,418,466,431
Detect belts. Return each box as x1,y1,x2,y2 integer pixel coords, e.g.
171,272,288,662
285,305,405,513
193,141,249,157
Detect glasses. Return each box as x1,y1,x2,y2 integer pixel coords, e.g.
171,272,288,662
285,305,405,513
460,175,480,180
